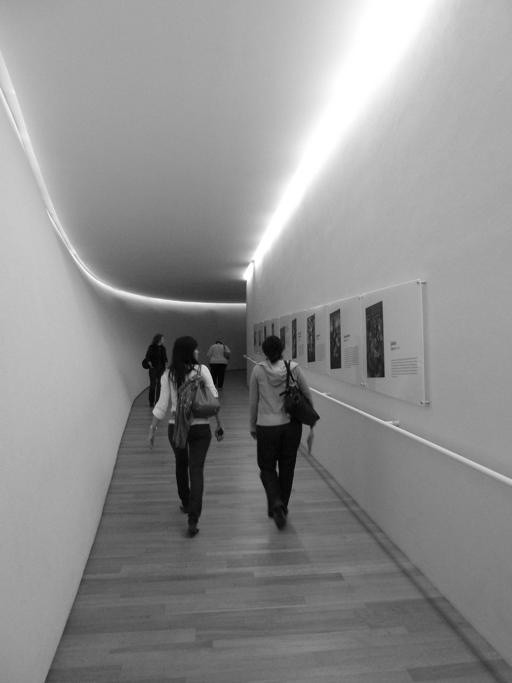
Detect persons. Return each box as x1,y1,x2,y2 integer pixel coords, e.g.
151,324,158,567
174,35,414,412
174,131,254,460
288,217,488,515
207,335,231,389
248,334,317,531
146,334,224,538
141,333,168,406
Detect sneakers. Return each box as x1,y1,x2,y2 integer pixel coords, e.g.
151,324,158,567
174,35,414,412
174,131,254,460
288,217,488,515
267,498,289,532
178,504,201,539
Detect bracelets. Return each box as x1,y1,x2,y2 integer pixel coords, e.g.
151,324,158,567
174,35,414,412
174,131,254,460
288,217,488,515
150,423,158,430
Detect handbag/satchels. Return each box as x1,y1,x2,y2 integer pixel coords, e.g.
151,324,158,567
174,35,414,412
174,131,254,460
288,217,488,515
184,371,221,420
280,383,320,426
142,359,149,370
224,351,231,360
